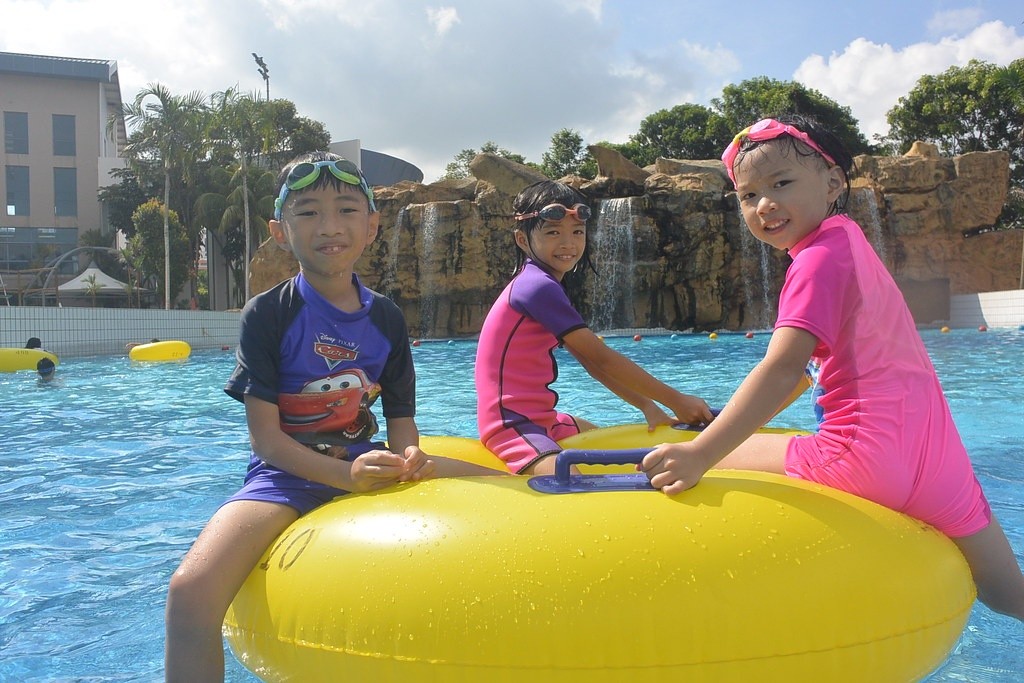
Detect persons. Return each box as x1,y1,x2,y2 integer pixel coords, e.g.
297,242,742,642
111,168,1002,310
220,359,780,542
125,335,159,351
159,141,438,680
24,337,48,355
470,174,715,488
633,108,1024,641
34,356,66,392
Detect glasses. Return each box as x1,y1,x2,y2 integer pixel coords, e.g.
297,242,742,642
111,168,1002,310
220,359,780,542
720,118,835,191
274,160,375,227
514,200,592,225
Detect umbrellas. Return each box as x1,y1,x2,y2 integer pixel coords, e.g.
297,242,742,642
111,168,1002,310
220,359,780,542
50,260,151,301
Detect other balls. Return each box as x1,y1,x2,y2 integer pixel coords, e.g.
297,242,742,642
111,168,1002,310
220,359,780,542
412,324,1024,347
222,345,229,350
